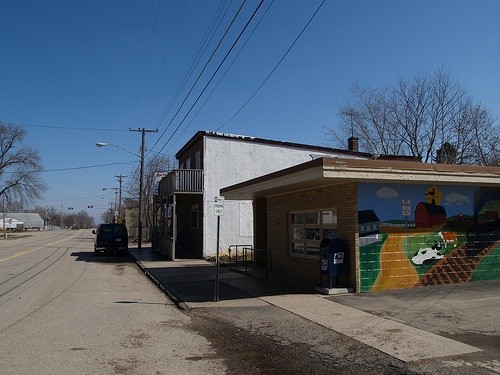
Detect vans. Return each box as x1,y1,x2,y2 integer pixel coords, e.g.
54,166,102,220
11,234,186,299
91,222,129,256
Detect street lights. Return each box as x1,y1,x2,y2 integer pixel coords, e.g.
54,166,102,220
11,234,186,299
94,128,145,249
101,187,119,221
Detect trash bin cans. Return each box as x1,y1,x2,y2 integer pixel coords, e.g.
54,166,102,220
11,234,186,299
318,237,351,290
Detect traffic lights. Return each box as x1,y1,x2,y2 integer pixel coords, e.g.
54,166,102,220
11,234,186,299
68,208,74,209
88,206,93,208
